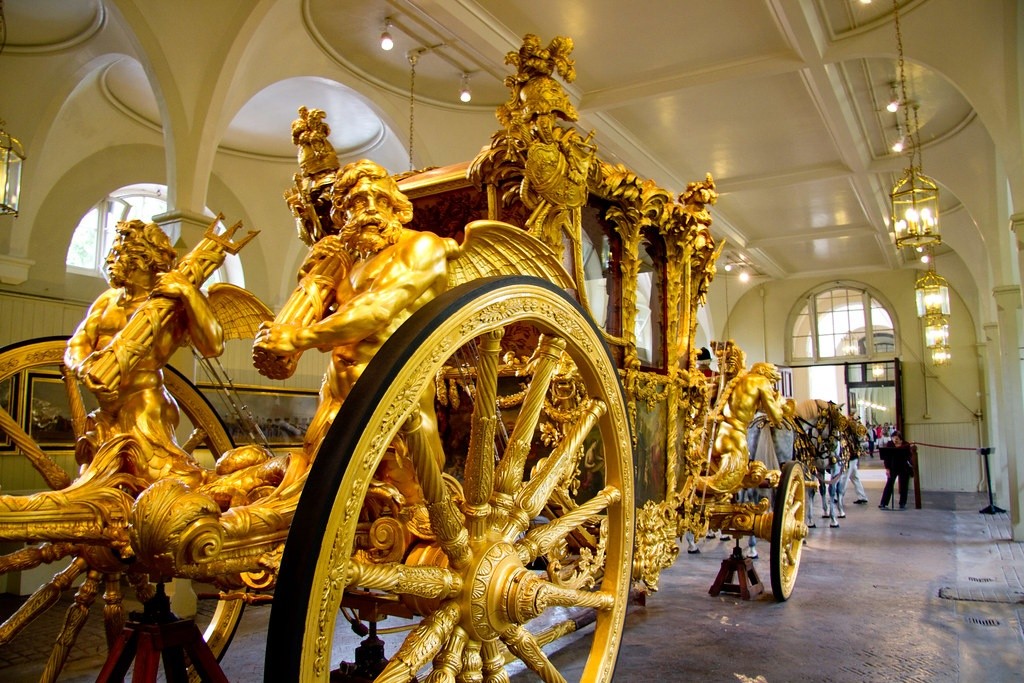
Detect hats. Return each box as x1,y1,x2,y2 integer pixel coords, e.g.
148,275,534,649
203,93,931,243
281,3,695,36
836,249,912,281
697,347,711,360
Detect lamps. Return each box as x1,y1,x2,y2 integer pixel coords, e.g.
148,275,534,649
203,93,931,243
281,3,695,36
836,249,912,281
378,17,396,51
840,331,859,357
915,268,950,368
740,264,749,281
886,93,901,113
725,256,734,272
0,119,28,220
871,364,885,379
459,71,473,103
889,167,942,248
890,135,906,154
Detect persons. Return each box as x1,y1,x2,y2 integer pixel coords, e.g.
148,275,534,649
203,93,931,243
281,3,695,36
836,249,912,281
696,347,720,379
865,422,897,458
258,160,447,507
831,438,868,503
717,360,788,467
878,430,914,508
64,221,223,477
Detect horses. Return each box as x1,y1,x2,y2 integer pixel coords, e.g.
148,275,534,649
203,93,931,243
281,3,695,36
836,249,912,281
685,400,869,559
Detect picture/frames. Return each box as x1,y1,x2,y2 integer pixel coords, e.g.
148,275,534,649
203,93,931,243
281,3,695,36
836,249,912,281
22,368,102,455
196,380,321,451
0,368,24,457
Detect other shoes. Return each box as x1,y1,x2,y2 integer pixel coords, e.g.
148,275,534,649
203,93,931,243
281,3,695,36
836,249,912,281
900,504,904,508
853,499,867,504
878,504,884,507
